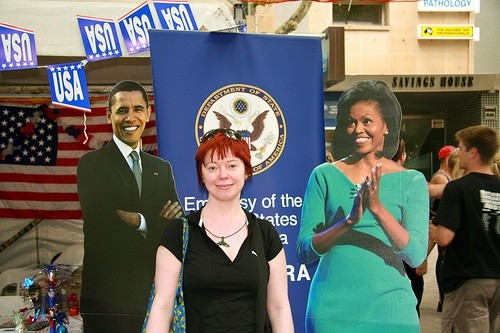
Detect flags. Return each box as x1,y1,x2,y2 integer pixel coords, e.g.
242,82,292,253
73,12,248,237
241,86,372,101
0,90,159,223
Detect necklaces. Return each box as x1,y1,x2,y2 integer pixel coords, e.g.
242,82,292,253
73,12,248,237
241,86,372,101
200,217,250,247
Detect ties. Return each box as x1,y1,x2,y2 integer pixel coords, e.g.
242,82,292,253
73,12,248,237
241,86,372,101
130,150,143,201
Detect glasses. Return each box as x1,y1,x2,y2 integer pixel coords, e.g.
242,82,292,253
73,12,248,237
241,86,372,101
200,128,242,142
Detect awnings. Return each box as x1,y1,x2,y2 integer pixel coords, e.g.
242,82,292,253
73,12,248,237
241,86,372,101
323,72,499,116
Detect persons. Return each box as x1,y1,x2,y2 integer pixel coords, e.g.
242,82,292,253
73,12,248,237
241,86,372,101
75,79,187,333
447,147,467,182
140,127,296,333
390,135,447,319
427,124,499,333
429,145,457,311
293,80,432,333
489,153,500,178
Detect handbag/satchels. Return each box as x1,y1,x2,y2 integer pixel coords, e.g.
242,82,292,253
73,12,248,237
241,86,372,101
141,217,188,333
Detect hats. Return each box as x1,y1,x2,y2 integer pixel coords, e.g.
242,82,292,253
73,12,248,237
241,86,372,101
438,145,456,159
